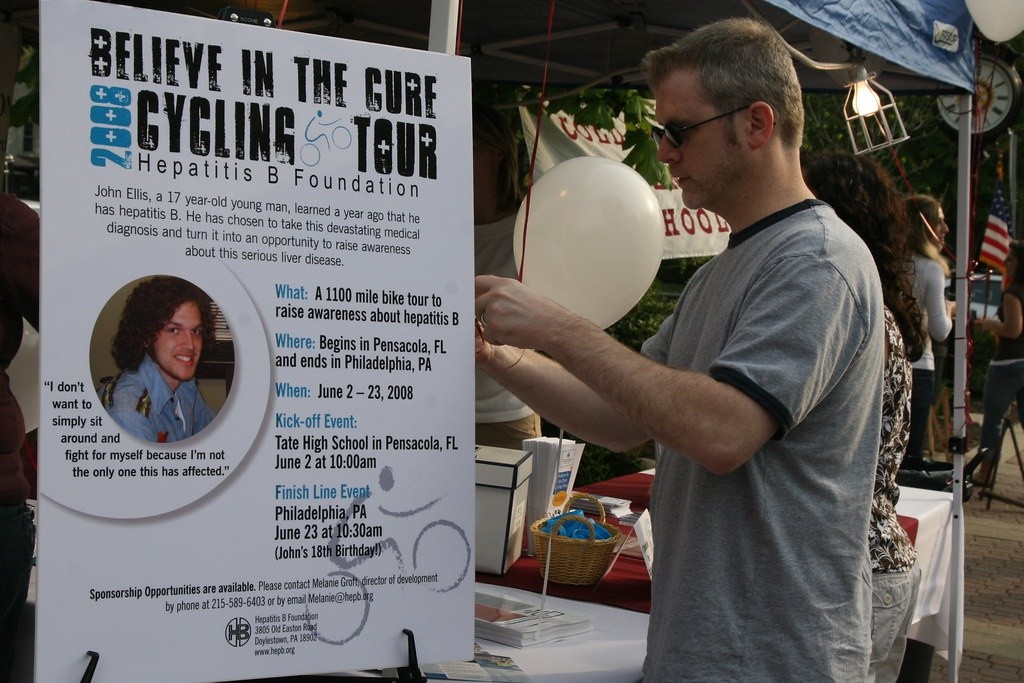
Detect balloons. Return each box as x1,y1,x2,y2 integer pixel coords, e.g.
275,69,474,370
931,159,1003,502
513,156,665,329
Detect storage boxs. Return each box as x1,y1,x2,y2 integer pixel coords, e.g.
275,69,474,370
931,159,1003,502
474,445,533,573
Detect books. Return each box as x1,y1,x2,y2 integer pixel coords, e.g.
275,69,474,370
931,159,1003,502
570,494,633,518
475,591,594,649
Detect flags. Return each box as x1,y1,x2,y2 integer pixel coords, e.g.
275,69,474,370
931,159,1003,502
981,186,1014,291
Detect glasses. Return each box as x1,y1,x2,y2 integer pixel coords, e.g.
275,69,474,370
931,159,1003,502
651,105,776,148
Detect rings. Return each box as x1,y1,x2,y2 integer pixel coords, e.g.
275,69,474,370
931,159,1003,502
480,314,488,325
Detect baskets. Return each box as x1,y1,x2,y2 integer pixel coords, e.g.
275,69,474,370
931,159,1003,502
528,493,621,584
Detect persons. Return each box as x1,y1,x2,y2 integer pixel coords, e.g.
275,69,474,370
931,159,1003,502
475,17,883,683
800,146,929,683
97,276,217,443
890,196,953,457
474,101,541,450
965,242,1024,487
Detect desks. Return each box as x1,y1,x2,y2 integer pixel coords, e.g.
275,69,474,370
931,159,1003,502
475,467,965,683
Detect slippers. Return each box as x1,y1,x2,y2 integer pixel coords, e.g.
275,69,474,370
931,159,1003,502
972,480,991,488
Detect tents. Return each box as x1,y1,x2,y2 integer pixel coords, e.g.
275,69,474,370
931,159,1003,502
424,0,977,683
